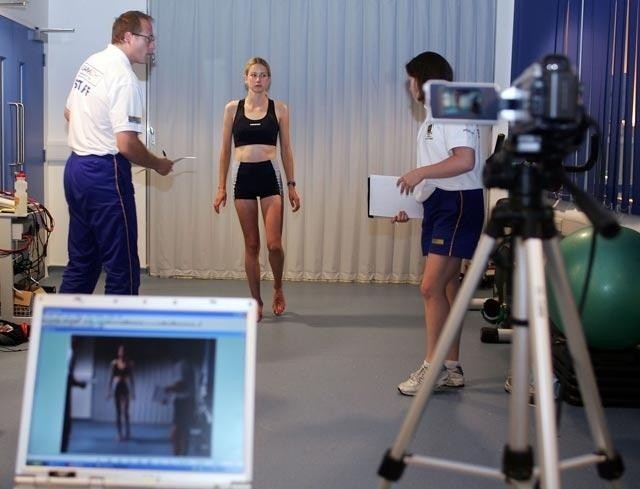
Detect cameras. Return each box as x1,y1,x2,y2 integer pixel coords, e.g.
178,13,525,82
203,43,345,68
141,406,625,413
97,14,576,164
423,51,586,133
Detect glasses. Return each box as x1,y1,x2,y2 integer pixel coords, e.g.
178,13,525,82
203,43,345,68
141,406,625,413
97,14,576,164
132,33,155,43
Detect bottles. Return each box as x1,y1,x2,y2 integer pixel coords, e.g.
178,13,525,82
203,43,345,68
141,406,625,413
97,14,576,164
14,173,28,216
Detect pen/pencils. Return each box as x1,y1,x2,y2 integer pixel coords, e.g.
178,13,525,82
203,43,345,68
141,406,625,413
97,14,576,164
163,151,167,158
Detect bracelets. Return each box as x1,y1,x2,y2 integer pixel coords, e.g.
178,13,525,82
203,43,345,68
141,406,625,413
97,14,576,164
287,181,296,186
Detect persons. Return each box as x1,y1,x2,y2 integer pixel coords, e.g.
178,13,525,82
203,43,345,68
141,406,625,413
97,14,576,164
390,51,485,396
214,57,300,324
57,349,86,452
154,347,197,456
106,345,137,443
58,10,173,295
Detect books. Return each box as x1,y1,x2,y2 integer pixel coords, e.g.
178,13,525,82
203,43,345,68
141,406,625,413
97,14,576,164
367,172,424,219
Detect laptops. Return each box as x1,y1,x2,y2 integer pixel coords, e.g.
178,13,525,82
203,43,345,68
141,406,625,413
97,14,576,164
11,293,260,489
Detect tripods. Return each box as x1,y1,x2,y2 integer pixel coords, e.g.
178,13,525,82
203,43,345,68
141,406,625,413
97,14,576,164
375,200,626,489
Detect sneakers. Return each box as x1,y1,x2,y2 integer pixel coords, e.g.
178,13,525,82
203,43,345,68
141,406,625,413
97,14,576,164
398,362,465,397
504,374,536,404
